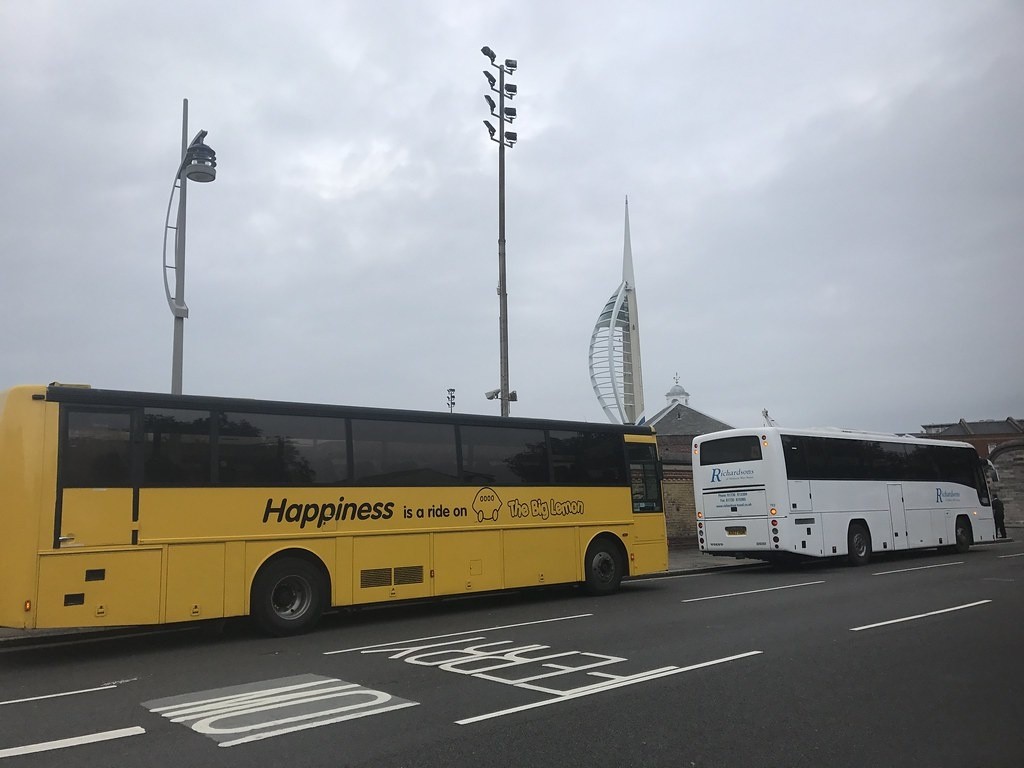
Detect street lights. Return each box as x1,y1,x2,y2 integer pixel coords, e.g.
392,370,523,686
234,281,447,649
481,45,518,417
162,98,217,438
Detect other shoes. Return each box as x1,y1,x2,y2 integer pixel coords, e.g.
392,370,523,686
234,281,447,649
1000,536,1006,538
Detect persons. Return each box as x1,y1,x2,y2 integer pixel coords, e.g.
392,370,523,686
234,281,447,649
992,494,1007,538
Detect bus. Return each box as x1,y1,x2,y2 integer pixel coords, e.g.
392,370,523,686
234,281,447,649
692,427,1002,567
0,382,669,638
